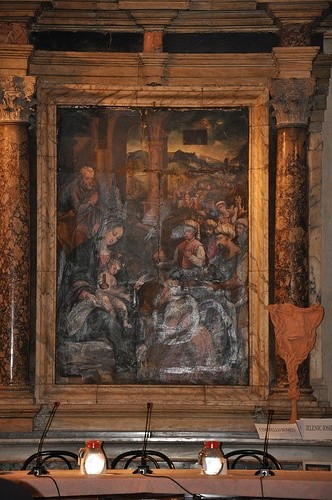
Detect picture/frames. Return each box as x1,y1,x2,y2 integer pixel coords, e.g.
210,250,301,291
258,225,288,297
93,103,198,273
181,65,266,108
33,84,268,403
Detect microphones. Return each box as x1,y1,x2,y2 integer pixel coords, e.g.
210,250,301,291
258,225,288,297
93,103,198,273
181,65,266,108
133,402,152,474
256,410,275,476
30,401,57,475
